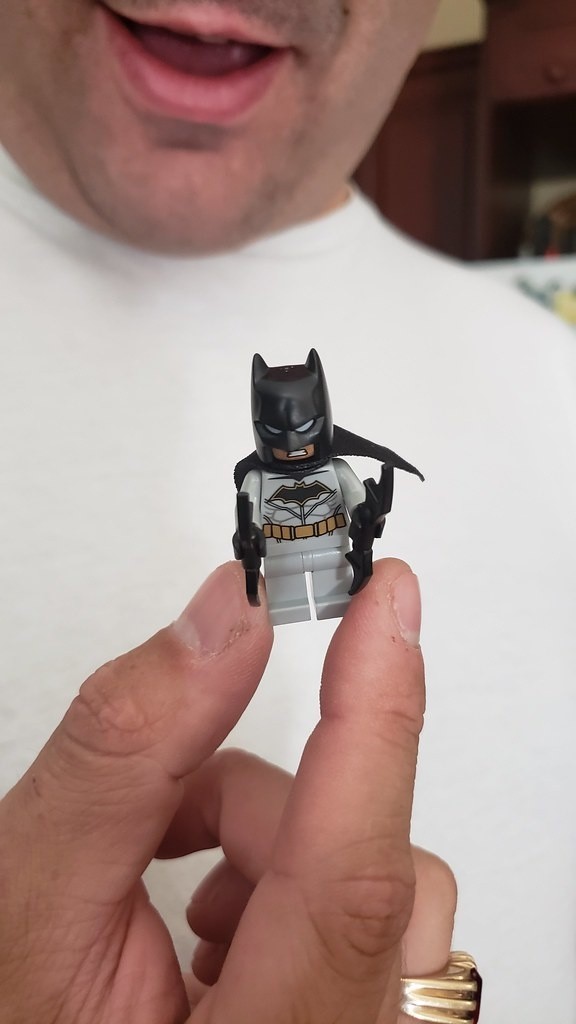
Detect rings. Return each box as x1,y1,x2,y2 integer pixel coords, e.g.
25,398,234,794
400,950,482,1024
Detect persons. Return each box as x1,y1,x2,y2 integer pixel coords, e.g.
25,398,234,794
231,348,425,626
0,0,576,1024
